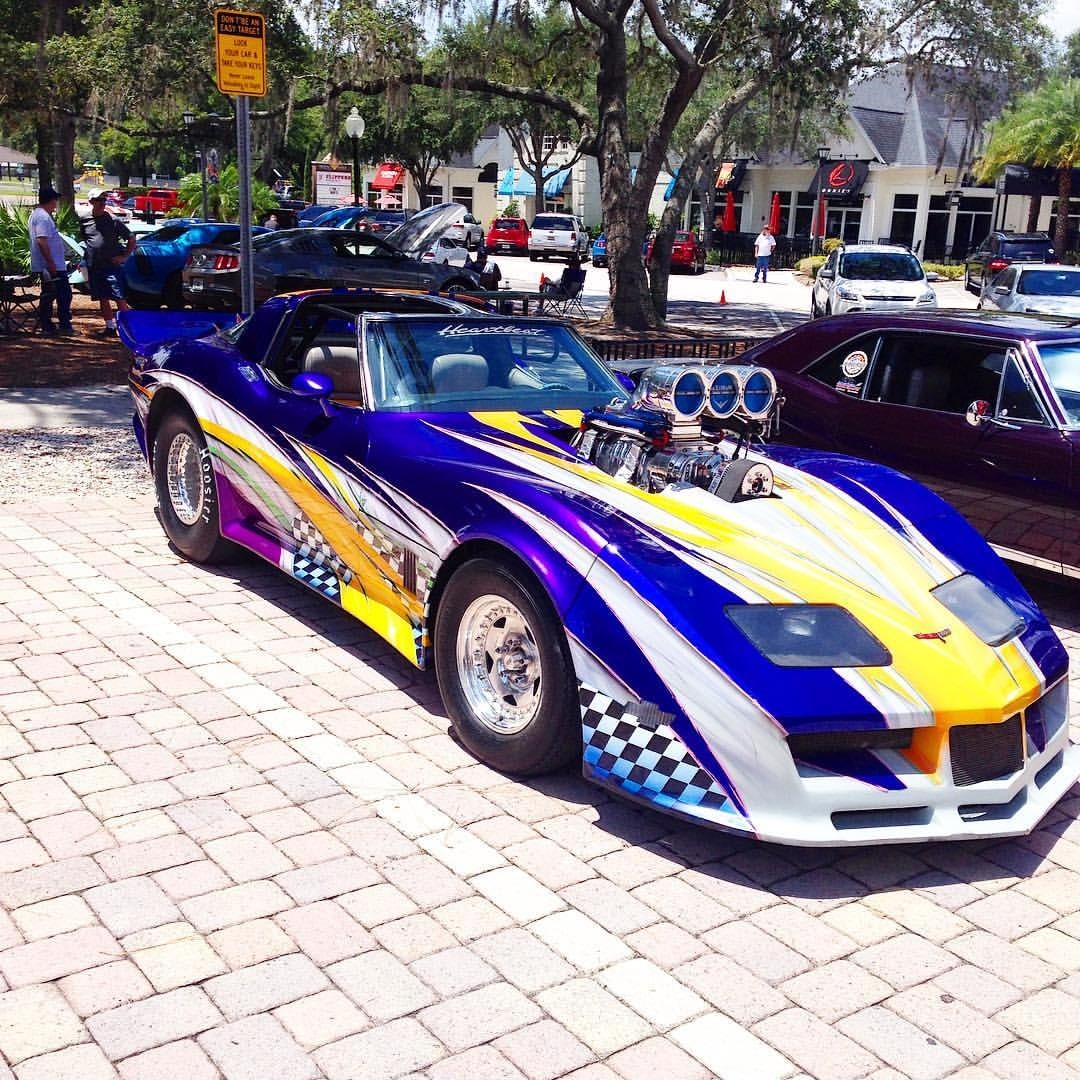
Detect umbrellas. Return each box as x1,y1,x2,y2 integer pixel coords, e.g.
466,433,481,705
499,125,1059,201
336,193,401,210
721,191,827,237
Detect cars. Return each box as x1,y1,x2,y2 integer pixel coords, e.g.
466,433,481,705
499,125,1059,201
96,188,188,218
591,231,707,274
631,310,1080,583
295,204,531,255
977,264,1080,319
69,203,503,313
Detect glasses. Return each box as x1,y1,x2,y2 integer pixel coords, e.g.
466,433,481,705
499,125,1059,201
94,197,106,202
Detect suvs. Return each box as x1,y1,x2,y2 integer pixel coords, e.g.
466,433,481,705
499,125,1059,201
528,212,592,262
963,229,1063,293
811,243,938,320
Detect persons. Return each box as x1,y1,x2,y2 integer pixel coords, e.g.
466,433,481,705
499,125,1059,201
532,256,585,314
463,246,501,292
752,224,776,283
28,167,135,340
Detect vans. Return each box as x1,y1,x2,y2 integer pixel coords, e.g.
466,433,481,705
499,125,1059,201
279,198,311,216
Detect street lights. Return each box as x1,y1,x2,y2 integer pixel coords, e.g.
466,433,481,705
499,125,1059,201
345,106,367,255
182,111,220,223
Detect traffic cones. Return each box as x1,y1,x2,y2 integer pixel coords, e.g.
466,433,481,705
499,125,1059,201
540,272,545,283
719,290,729,305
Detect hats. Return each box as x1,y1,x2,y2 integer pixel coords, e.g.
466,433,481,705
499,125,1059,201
88,188,108,201
39,187,62,202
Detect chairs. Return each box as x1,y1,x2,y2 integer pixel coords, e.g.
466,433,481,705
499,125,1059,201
432,354,489,393
304,346,361,400
0,273,40,334
545,268,588,320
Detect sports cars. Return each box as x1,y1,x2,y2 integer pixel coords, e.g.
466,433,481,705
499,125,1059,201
112,285,1079,848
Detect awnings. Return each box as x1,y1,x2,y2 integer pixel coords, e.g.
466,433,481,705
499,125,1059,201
694,160,748,193
372,165,406,190
498,168,573,197
807,160,870,197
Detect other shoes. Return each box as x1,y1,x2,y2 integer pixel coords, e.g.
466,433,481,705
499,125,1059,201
60,329,79,336
43,329,56,335
97,327,117,337
762,277,766,282
752,277,759,282
